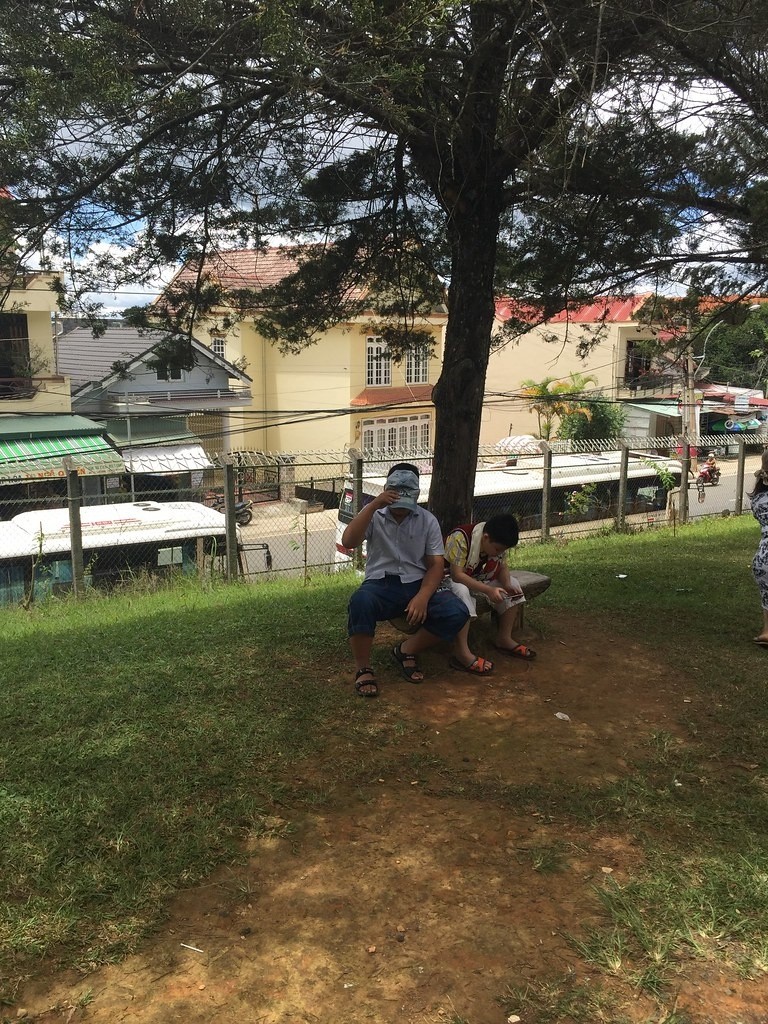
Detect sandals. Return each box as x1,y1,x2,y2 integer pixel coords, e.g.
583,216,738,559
391,639,423,684
355,668,378,697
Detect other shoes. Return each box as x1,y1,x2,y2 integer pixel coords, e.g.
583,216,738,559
710,479,713,482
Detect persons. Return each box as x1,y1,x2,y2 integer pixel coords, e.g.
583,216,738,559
706,453,716,481
746,450,768,644
341,463,470,697
442,512,537,676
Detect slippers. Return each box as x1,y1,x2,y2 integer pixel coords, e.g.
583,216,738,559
752,636,768,645
448,656,495,676
497,644,537,661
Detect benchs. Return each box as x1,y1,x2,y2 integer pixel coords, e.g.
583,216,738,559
384,569,551,655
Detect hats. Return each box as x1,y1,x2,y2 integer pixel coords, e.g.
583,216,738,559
754,450,768,485
385,469,419,512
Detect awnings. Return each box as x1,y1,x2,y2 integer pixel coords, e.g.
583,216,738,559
0,434,126,486
121,444,215,476
627,400,728,418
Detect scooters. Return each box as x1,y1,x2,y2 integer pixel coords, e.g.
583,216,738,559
696,462,722,486
209,492,254,526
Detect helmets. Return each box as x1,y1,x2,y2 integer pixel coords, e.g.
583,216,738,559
708,453,714,457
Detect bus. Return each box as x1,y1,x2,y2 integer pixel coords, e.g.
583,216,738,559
330,448,706,575
0,500,274,612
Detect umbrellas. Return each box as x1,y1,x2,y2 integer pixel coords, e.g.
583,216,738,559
712,418,763,430
